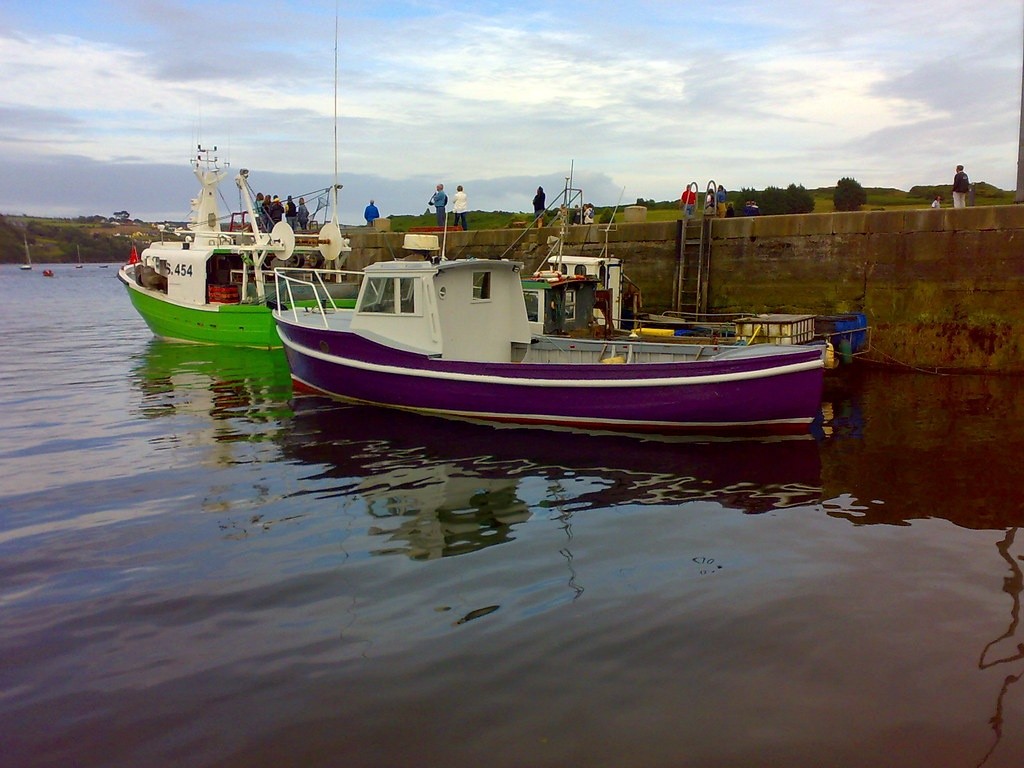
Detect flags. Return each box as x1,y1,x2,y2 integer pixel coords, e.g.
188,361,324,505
127,246,137,264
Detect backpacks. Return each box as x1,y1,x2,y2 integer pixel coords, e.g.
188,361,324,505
444,194,448,206
589,208,594,218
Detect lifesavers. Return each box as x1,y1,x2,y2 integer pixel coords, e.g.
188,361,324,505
209,284,241,303
531,271,562,283
562,275,585,279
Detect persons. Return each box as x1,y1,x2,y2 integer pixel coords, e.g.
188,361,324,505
268,195,284,233
261,195,271,233
284,195,297,230
950,165,970,208
533,186,545,228
364,200,379,226
932,195,941,208
705,189,715,211
582,204,587,224
743,201,761,217
584,203,595,224
724,203,734,217
717,185,727,218
452,184,469,230
679,185,695,218
573,205,581,224
254,192,264,225
298,197,309,230
434,183,445,226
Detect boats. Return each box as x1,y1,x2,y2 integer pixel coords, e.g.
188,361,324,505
99,265,109,268
43,269,54,276
520,256,866,368
117,144,389,350
270,235,834,445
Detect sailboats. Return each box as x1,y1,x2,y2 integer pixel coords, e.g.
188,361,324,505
20,234,32,269
76,245,83,268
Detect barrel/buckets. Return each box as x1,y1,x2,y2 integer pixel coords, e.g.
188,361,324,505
208,283,240,303
